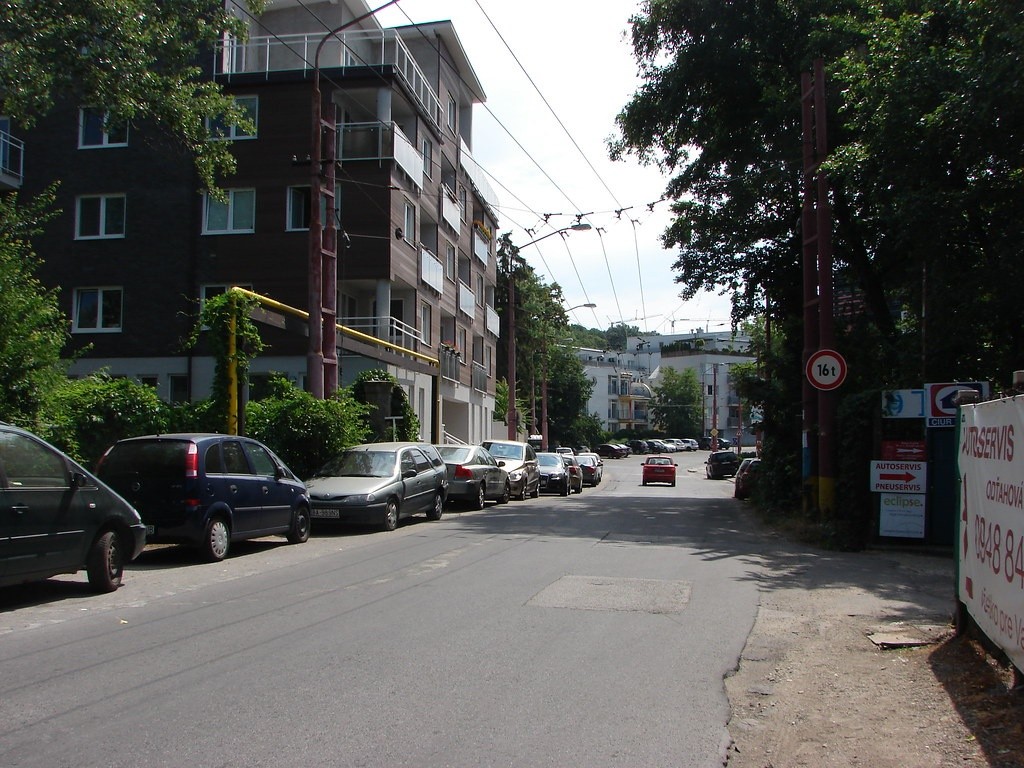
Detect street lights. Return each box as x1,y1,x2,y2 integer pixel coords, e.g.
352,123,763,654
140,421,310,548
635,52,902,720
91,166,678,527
507,223,594,441
539,303,596,452
701,363,725,437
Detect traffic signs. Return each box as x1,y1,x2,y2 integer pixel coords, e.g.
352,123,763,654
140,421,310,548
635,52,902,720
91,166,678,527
881,438,928,462
869,459,928,494
926,417,956,428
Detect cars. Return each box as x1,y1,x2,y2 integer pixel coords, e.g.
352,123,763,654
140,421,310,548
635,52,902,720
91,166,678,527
573,446,604,487
704,451,739,479
85,432,313,562
644,438,700,454
0,422,148,593
560,454,584,494
734,457,763,501
479,440,541,501
534,452,572,496
300,441,450,532
612,443,632,457
554,447,574,456
624,440,649,454
641,456,678,487
592,444,628,459
430,444,511,510
